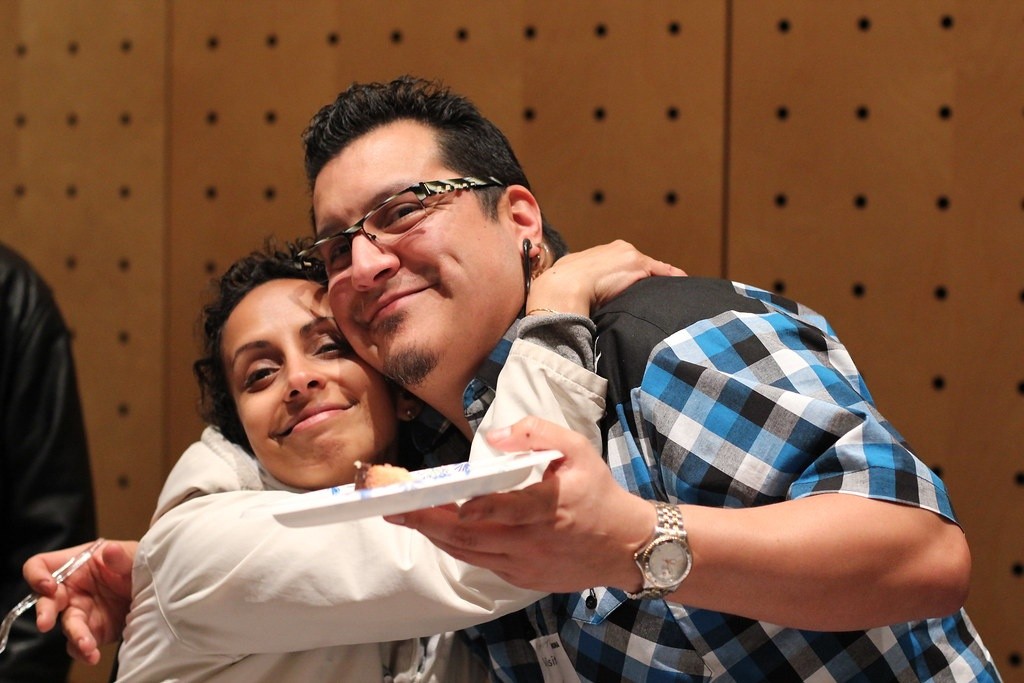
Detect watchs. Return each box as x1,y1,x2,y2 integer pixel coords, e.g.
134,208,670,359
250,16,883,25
624,497,693,602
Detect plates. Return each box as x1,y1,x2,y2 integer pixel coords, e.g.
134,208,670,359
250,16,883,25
266,451,563,528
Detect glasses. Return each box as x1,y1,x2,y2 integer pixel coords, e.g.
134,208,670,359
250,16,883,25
294,175,508,284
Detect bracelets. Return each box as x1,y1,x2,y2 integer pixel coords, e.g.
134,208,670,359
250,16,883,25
525,307,558,315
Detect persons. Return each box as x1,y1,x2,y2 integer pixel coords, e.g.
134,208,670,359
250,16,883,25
114,230,688,683
23,74,1006,683
0,240,100,683
